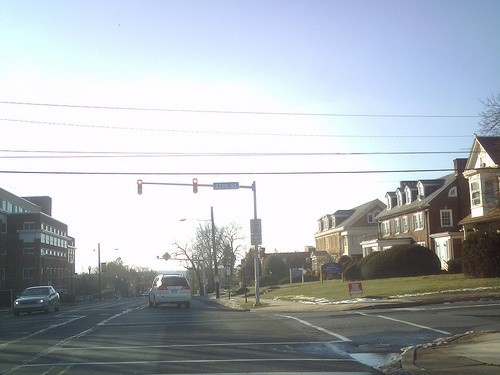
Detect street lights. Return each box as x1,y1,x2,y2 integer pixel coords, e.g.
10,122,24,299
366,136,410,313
97,242,120,301
88,266,92,302
179,205,220,300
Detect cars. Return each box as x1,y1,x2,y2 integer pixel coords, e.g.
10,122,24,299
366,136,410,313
13,285,62,316
148,273,192,309
142,292,149,297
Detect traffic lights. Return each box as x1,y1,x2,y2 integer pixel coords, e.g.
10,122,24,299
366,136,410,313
137,179,143,195
193,178,199,194
260,248,265,258
